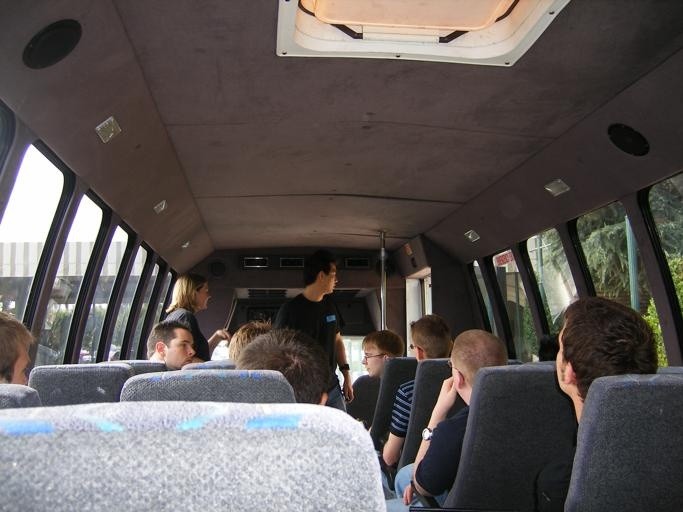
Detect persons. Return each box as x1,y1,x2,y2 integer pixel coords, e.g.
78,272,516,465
0,307,37,389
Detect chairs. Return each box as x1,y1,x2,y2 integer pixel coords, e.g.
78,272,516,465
1,359,681,512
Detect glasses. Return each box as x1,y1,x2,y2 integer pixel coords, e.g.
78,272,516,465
409,344,425,352
363,353,388,360
447,360,460,372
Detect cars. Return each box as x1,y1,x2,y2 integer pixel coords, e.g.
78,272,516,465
73,347,134,362
